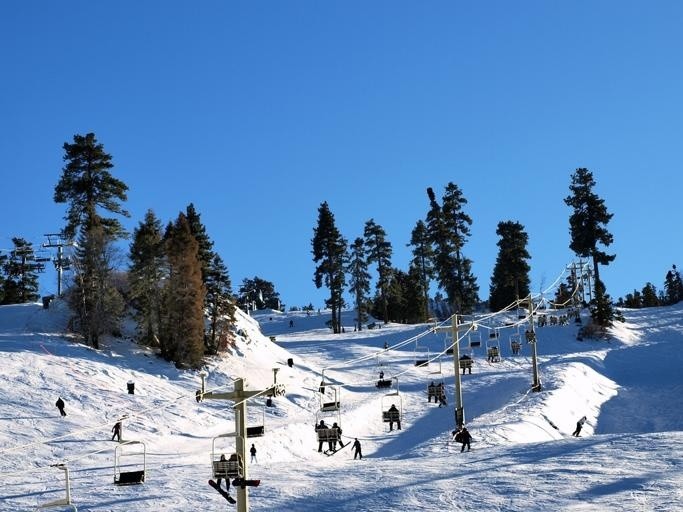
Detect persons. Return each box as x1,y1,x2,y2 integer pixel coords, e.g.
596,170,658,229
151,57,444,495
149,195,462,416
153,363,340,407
54,396,66,418
110,421,120,443
486,345,494,363
289,320,293,329
427,380,439,404
377,370,384,382
460,353,473,374
529,329,536,344
383,341,388,349
305,309,311,320
493,346,500,363
228,452,246,489
511,338,518,355
330,422,344,452
436,382,447,406
571,416,586,438
536,307,574,327
387,403,401,433
194,387,202,406
314,419,333,452
249,443,258,464
459,427,473,452
350,437,362,460
214,454,231,499
525,329,530,344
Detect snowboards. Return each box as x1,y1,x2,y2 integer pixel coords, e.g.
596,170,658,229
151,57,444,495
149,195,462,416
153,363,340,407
312,439,350,456
207,479,261,505
